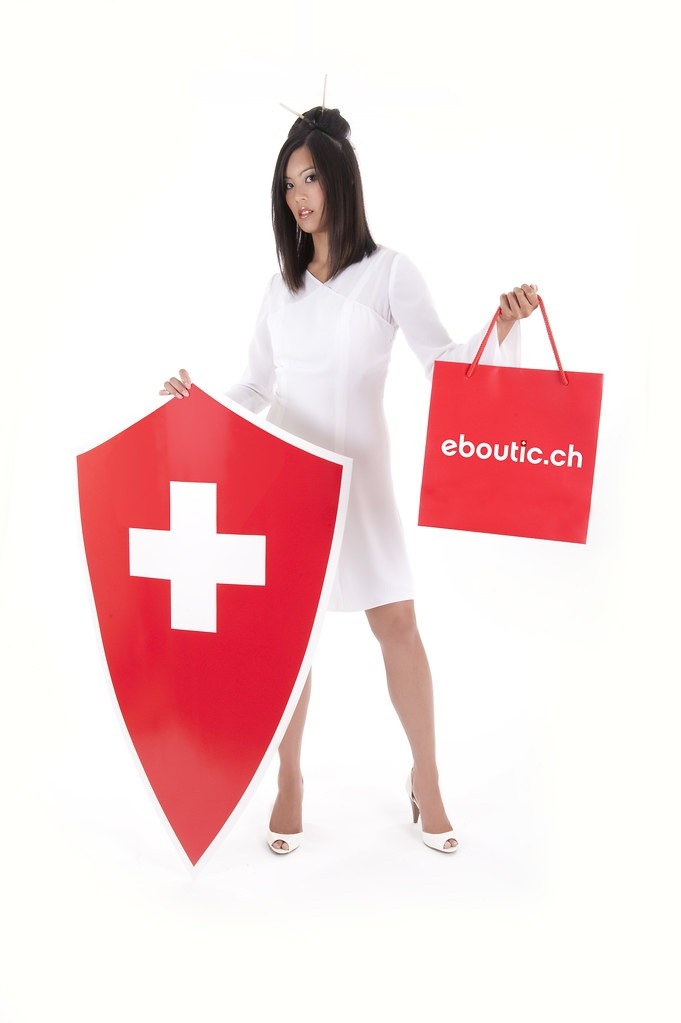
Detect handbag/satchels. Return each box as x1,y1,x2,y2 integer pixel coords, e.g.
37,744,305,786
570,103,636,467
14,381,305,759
417,292,605,547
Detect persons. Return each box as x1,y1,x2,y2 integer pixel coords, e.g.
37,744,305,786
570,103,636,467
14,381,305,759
156,107,540,854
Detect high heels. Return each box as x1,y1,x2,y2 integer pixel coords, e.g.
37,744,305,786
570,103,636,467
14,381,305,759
411,771,459,855
267,776,304,855
